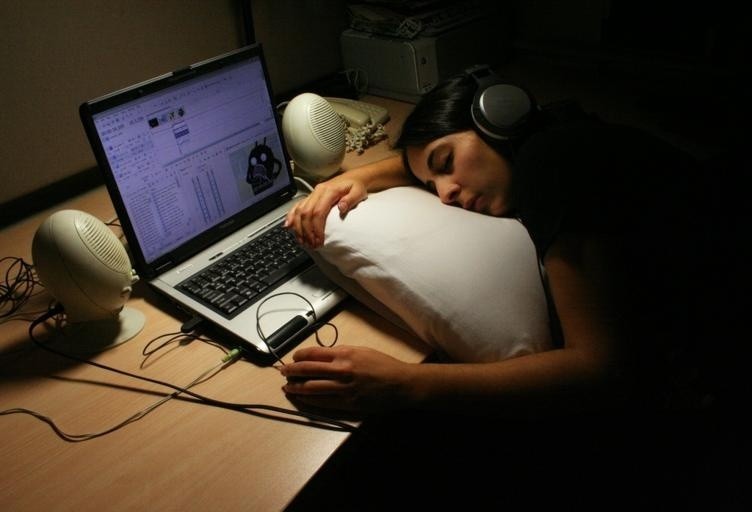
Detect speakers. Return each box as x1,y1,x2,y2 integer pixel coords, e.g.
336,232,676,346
282,92,346,194
31,211,139,328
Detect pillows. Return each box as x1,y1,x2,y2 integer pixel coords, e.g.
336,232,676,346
313,185,554,364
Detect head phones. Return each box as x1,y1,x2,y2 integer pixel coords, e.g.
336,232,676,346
454,65,537,142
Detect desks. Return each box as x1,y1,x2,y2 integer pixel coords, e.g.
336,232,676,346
1,93,433,511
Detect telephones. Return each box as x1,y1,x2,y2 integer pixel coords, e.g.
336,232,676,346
322,96,391,154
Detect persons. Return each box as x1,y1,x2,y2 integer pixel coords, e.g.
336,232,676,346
279,63,618,408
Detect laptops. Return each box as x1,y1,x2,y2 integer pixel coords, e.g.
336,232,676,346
80,42,351,364
